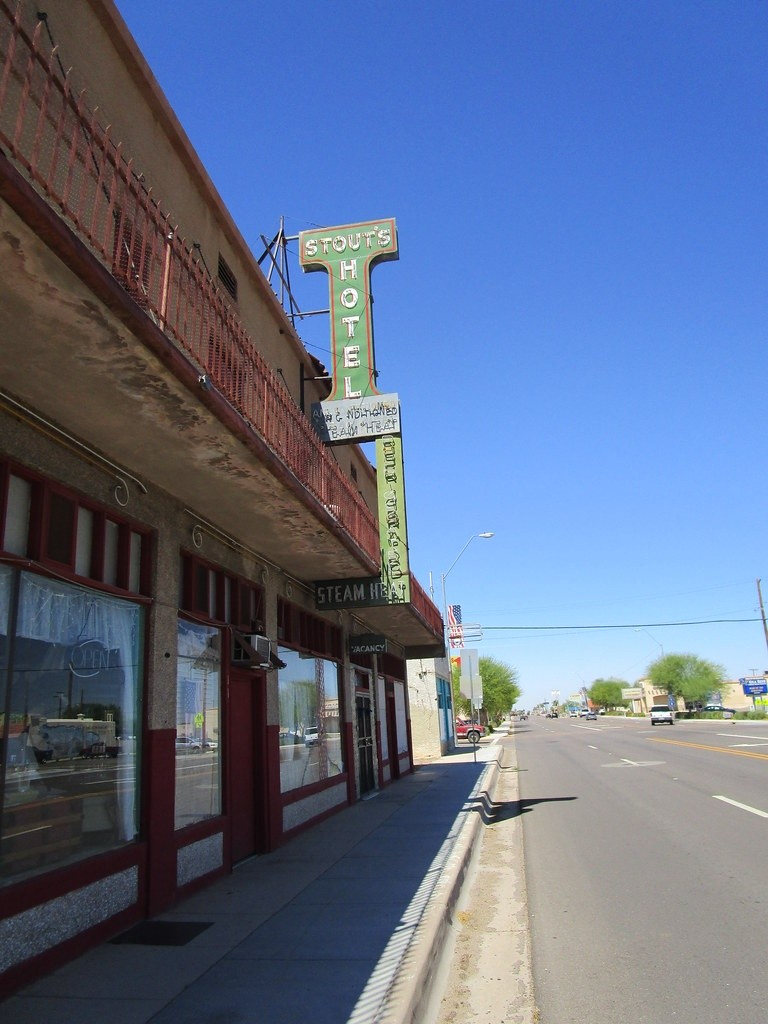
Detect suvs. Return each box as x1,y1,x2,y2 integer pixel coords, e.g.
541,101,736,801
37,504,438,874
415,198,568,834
304,727,321,746
455,716,485,743
579,709,589,717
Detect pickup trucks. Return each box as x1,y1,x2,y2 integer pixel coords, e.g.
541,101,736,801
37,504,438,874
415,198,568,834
79,742,122,759
649,705,673,725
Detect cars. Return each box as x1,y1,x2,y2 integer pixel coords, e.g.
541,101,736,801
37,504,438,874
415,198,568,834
198,738,218,752
24,747,47,765
279,733,299,744
570,712,577,717
702,705,736,714
175,736,202,753
586,713,597,720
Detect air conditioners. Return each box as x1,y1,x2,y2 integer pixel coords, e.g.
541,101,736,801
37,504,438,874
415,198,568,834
231,635,272,668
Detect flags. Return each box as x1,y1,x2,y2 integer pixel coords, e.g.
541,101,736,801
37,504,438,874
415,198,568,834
448,606,464,648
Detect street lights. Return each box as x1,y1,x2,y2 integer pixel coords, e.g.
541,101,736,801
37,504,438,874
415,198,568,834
441,532,496,746
54,691,67,719
635,628,664,657
